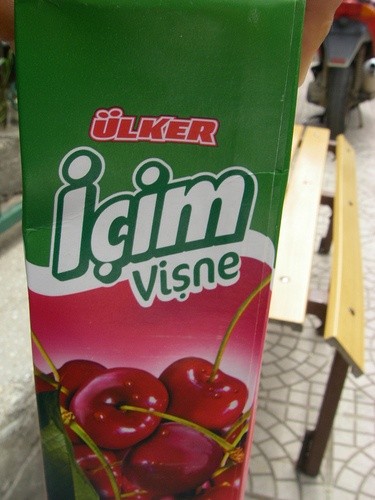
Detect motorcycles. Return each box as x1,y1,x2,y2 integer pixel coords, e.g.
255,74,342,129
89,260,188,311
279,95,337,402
305,1,375,139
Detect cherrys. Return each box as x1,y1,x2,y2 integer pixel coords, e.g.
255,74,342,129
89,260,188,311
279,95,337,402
31,275,272,499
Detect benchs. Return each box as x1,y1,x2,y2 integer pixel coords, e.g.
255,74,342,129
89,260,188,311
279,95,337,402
268,124,363,477
